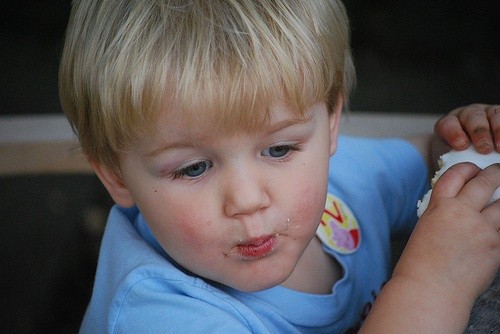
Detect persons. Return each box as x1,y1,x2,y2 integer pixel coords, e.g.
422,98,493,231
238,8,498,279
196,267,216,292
58,1,500,334
